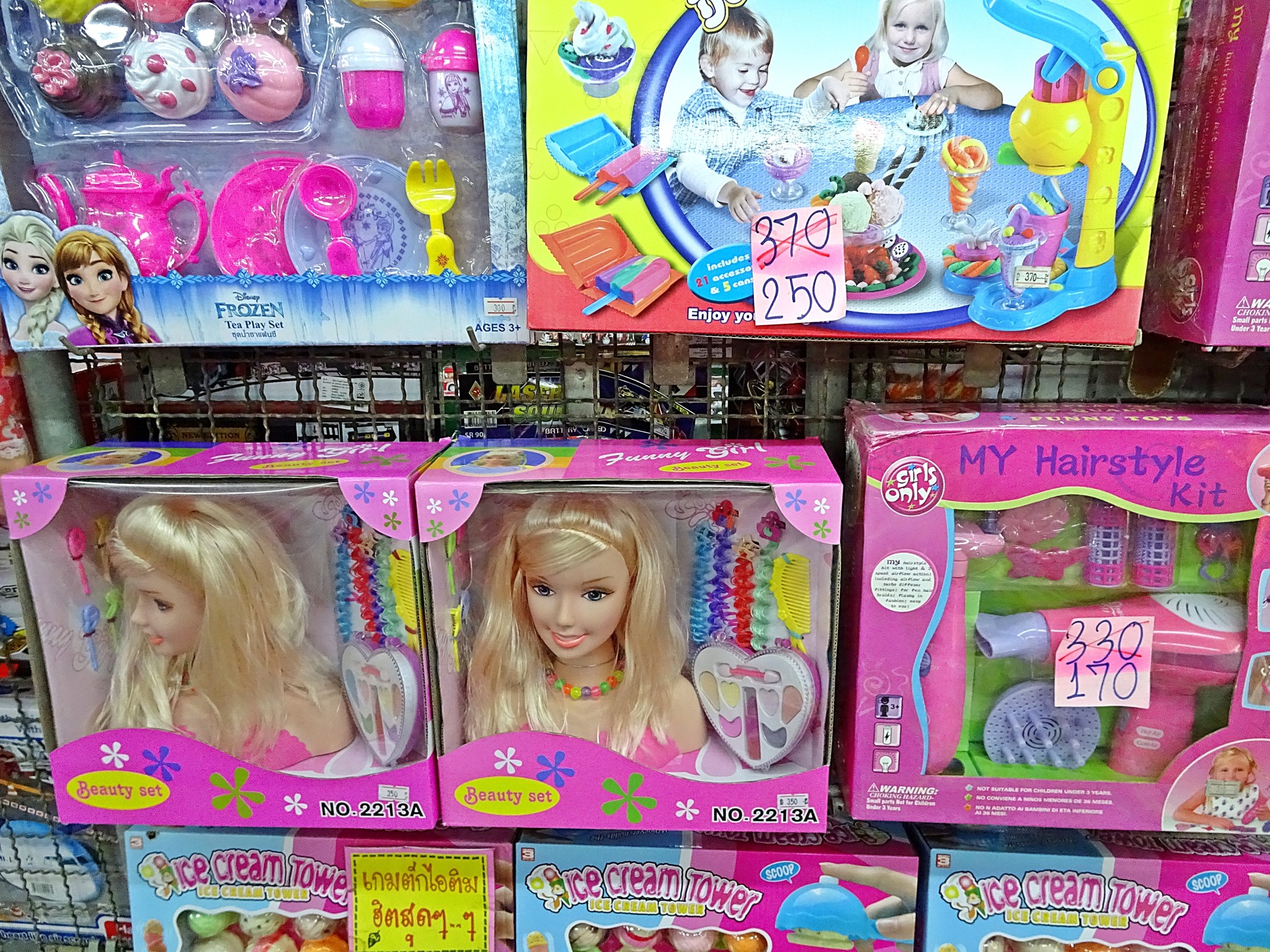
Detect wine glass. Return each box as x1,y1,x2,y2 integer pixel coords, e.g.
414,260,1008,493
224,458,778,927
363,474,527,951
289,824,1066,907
989,223,1048,311
939,155,992,233
764,150,812,201
558,32,636,98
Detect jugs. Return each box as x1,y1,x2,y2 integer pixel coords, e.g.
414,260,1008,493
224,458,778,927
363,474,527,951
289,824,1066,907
36,150,210,277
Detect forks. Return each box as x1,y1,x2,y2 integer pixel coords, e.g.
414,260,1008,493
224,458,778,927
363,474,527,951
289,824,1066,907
405,158,461,276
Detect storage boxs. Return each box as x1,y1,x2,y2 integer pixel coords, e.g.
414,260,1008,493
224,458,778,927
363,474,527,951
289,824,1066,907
0,0,1270,952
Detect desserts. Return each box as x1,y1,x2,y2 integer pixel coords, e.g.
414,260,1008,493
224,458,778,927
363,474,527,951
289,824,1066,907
214,0,289,24
122,31,213,120
35,0,105,24
31,35,123,121
122,0,201,23
216,33,304,125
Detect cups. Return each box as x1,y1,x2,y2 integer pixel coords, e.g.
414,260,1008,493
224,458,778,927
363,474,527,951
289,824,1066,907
852,127,884,174
1019,199,1072,267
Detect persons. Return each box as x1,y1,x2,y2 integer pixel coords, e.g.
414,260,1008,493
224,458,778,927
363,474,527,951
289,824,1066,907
492,861,518,949
92,493,355,770
793,0,1003,116
667,5,849,223
463,492,707,755
820,862,917,952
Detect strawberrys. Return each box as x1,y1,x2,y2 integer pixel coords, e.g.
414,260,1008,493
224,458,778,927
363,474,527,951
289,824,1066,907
845,247,892,283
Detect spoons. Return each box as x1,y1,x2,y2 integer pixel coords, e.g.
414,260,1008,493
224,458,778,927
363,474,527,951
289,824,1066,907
299,163,362,277
855,46,869,73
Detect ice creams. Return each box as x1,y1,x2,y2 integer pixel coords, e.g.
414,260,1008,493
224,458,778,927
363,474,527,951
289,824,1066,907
851,116,886,175
999,178,1068,295
810,145,927,248
765,143,812,178
940,136,989,214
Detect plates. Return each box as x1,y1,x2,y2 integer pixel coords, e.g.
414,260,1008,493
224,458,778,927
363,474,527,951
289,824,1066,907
284,155,432,274
846,238,927,299
211,157,315,275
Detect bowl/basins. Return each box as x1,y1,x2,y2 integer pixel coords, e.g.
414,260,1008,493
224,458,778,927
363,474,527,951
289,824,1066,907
843,213,903,247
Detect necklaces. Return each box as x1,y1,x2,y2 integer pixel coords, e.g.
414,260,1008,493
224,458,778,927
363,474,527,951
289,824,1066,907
555,657,616,668
544,665,625,698
